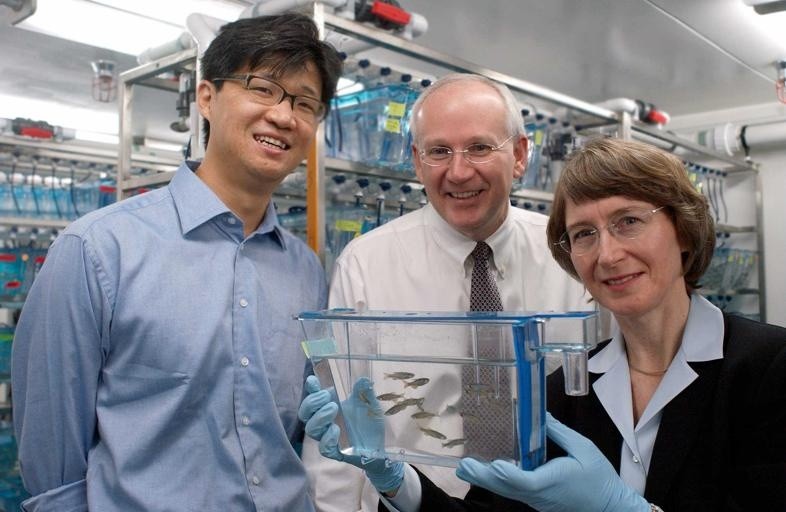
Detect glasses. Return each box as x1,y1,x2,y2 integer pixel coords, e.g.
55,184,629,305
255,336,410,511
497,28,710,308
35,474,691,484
418,136,514,166
553,207,663,256
209,73,326,124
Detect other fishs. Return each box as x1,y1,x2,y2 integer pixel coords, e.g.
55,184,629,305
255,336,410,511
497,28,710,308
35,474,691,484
356,371,507,449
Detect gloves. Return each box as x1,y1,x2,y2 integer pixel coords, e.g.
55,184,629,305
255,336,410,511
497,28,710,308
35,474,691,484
298,374,404,493
456,411,652,512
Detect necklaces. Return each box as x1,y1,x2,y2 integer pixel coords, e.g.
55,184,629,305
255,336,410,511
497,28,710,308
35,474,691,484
629,361,668,376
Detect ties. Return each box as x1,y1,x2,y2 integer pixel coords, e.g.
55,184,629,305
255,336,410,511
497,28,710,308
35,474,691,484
461,241,515,462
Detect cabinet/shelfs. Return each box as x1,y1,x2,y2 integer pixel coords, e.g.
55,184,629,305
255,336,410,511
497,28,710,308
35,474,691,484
116,0,768,327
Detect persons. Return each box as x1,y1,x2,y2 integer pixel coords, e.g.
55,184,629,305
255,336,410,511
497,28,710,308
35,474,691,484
299,68,617,512
296,133,784,512
8,11,343,511
